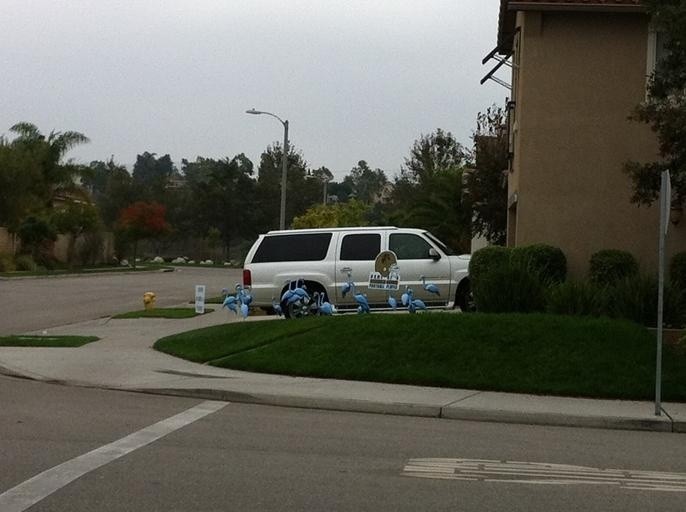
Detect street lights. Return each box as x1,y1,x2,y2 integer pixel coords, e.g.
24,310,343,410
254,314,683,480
306,174,328,206
245,108,289,230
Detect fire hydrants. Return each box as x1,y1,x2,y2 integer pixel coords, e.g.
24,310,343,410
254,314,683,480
141,291,156,311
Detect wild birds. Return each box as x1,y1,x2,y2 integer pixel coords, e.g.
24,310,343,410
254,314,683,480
342,272,352,309
351,281,370,314
271,277,332,319
388,284,426,313
221,283,255,321
419,275,441,307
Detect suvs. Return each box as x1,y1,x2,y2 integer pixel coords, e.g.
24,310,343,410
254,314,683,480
243,226,475,320
